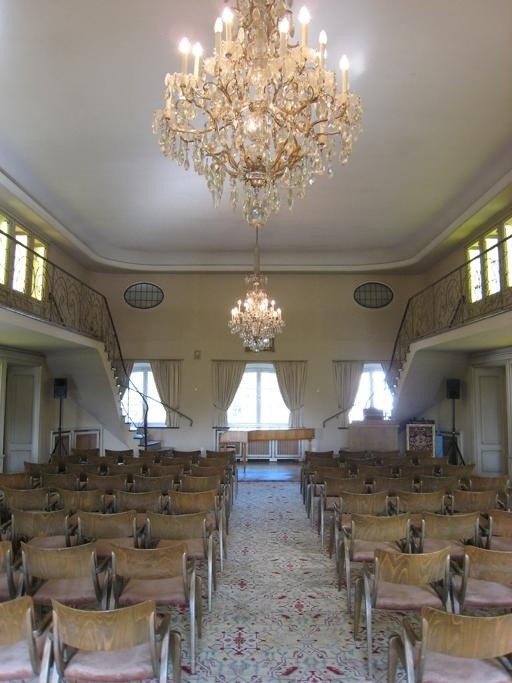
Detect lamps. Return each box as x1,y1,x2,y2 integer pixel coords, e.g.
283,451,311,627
147,0,368,224
224,223,286,353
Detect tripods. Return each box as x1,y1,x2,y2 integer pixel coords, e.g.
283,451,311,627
49,398,67,464
444,399,466,466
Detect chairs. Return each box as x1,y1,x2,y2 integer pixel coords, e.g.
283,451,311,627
300,446,512,682
0,444,238,682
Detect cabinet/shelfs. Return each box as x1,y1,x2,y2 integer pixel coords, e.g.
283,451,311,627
214,428,304,460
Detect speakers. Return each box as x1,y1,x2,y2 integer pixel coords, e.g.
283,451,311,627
54,378,67,398
447,379,460,398
